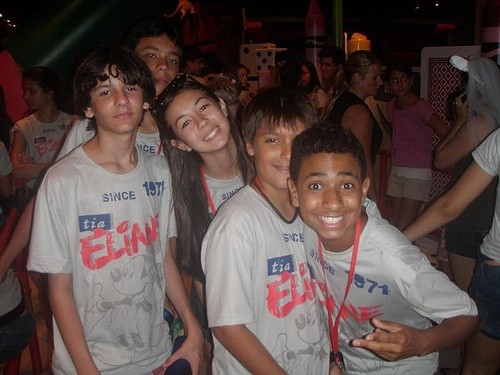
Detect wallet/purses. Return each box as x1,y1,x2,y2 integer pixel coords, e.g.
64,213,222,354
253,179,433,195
165,336,193,375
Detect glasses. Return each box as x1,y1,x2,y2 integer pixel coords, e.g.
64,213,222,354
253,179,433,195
148,73,202,130
388,75,409,84
